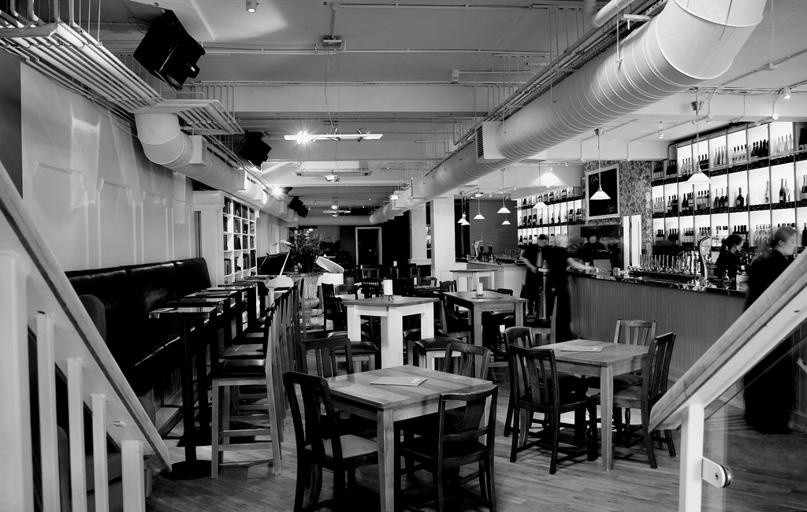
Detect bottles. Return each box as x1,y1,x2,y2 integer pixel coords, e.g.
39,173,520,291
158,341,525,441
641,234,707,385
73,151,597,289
653,186,747,214
696,255,704,276
652,161,677,180
680,134,792,175
765,181,769,205
779,176,806,203
522,190,571,206
655,222,807,250
522,208,582,227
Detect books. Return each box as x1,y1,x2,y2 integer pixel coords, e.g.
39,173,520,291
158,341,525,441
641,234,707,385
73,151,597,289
559,343,603,353
369,376,428,387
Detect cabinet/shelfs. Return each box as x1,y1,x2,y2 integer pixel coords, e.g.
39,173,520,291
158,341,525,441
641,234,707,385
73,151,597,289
220,189,257,276
512,188,582,250
646,131,807,233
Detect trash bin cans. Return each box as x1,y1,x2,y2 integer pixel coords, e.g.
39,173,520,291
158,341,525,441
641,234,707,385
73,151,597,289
346,277,353,288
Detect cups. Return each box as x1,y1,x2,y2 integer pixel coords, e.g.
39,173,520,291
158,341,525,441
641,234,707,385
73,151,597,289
494,247,522,260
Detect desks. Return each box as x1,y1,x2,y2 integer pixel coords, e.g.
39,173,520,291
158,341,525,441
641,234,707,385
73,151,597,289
532,338,661,470
319,365,493,512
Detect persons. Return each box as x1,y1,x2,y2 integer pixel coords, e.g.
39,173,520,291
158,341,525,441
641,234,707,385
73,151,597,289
545,235,592,342
520,233,549,315
741,227,798,433
714,235,744,278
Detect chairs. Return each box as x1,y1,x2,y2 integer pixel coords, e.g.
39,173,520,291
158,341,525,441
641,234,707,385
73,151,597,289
147,269,306,483
298,334,378,439
321,270,558,371
280,370,379,511
399,384,497,512
503,326,555,437
695,237,719,280
401,342,492,491
585,331,677,469
509,344,598,474
588,319,657,443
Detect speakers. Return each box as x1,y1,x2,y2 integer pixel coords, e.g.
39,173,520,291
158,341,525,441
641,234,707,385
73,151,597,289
132,10,205,91
238,131,272,167
286,197,308,217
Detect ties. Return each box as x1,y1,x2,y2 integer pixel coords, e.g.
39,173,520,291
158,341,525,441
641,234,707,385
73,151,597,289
536,248,544,268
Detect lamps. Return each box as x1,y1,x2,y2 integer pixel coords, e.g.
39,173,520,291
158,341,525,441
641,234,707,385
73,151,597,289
324,171,343,186
447,64,464,86
687,99,710,183
389,189,399,202
454,127,614,225
780,86,795,103
242,0,261,15
330,203,340,210
